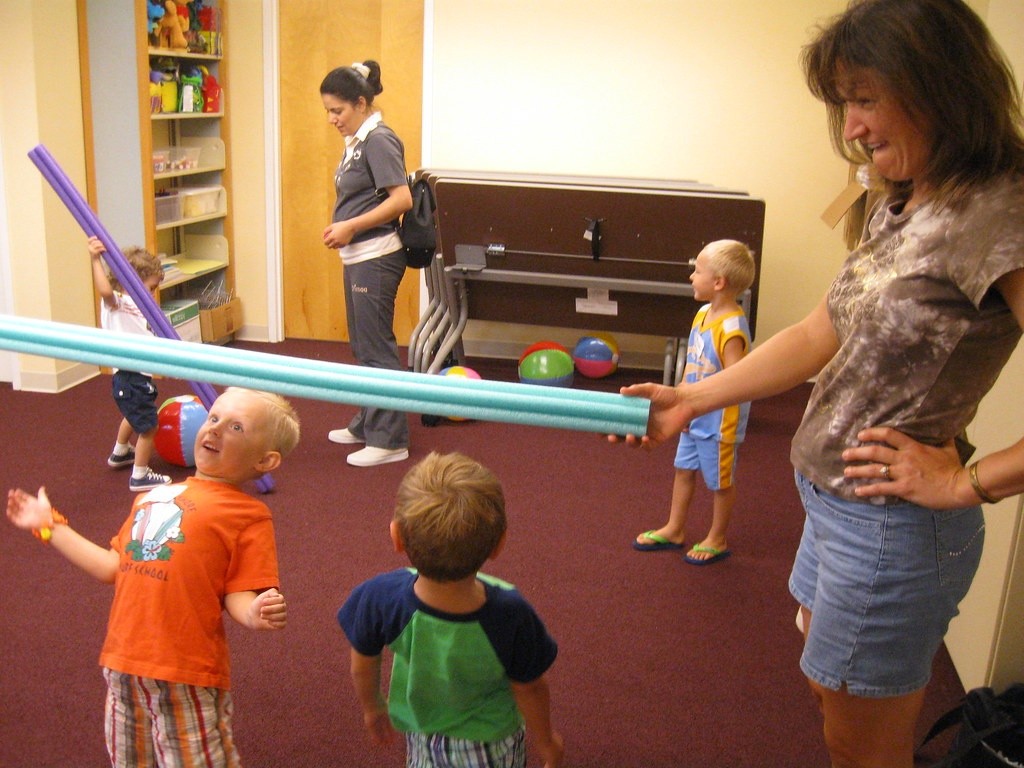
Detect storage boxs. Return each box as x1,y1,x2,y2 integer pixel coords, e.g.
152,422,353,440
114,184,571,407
153,187,223,226
150,146,201,174
160,298,244,344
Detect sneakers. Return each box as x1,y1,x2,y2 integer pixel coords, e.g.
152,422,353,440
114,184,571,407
329,428,364,445
108,442,137,467
128,467,170,491
347,442,410,467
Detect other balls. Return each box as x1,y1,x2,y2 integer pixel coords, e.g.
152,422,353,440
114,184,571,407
153,395,209,467
517,340,576,388
573,333,620,378
438,365,483,422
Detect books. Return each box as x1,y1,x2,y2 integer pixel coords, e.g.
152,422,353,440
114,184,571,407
155,252,184,287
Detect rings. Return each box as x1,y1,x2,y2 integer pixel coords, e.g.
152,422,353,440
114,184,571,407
881,463,892,478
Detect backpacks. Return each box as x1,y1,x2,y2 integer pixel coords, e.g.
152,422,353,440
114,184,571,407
367,131,435,268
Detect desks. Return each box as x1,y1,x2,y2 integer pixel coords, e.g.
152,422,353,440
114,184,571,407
406,168,765,427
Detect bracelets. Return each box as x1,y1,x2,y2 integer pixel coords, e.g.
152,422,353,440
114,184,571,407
31,508,70,548
968,460,1005,505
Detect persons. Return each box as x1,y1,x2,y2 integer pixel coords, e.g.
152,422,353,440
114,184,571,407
85,235,173,491
286,61,442,468
7,385,301,768
604,1,1024,768
632,240,755,565
337,452,556,768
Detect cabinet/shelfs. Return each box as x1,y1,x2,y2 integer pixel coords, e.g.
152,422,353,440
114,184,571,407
76,0,237,380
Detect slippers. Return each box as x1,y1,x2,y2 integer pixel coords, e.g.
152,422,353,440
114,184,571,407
634,528,683,550
685,535,730,564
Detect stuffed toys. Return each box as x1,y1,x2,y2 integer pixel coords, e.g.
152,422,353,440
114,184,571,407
147,0,221,113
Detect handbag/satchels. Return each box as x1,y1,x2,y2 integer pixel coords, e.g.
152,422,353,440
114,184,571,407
916,682,1024,768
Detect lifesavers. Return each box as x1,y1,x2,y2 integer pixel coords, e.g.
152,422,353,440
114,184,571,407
25,144,275,496
1,312,651,441
